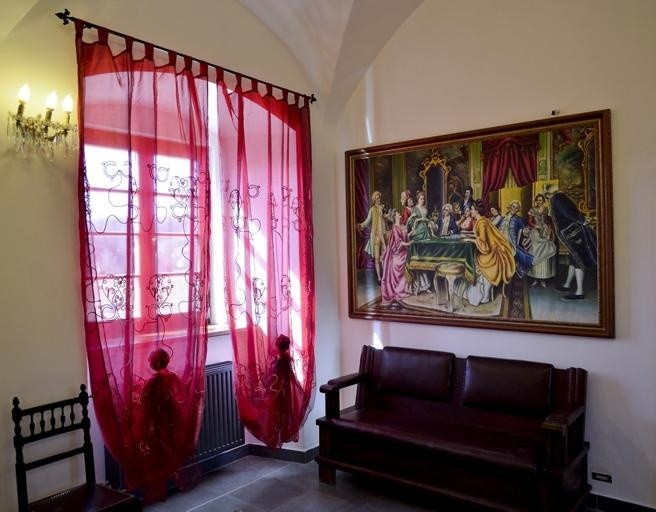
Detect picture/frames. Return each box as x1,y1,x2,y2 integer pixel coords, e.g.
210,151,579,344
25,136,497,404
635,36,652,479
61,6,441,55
346,108,615,339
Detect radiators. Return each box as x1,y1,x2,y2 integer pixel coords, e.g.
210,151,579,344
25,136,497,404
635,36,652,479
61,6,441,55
105,359,247,491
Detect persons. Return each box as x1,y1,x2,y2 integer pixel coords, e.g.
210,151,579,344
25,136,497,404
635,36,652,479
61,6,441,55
138,348,185,476
259,333,291,449
356,185,598,310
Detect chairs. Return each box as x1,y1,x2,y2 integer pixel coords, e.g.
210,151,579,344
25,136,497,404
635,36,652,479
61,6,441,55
11,384,144,511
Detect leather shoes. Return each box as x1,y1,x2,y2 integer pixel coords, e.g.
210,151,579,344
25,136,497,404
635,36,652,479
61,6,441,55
553,287,571,293
560,294,585,302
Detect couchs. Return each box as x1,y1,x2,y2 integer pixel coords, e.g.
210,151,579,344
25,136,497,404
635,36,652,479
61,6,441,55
316,345,590,512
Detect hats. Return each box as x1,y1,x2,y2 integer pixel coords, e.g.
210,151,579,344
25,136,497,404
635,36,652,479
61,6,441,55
416,190,426,199
400,190,412,206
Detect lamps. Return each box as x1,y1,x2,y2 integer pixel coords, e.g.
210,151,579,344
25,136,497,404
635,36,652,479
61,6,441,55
7,83,80,162
